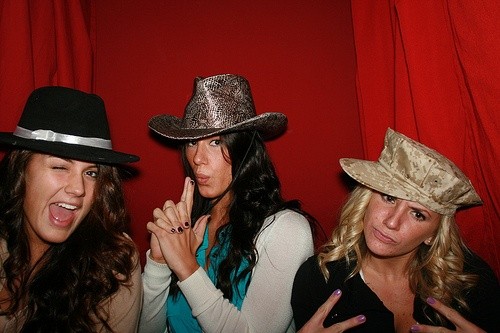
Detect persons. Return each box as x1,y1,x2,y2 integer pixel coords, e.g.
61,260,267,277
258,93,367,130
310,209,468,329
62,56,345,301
291,126,500,333
138,73,315,333
0,85,144,333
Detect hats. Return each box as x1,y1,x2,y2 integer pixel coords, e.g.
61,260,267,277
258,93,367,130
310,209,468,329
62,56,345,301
339,127,484,217
0,86,140,164
147,73,288,141
